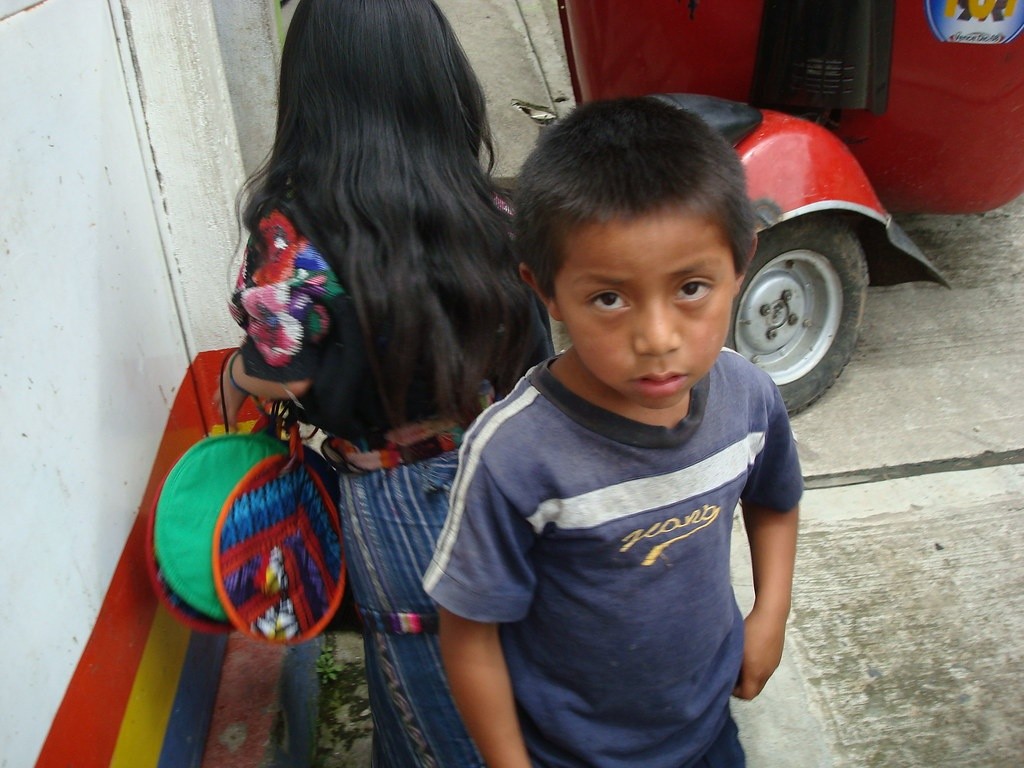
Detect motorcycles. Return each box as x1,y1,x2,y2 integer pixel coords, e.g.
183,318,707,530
554,0,1024,427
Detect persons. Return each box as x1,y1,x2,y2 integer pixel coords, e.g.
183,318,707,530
423,97,804,768
214,0,555,768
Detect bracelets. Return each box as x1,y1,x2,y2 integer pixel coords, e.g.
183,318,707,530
229,351,250,395
